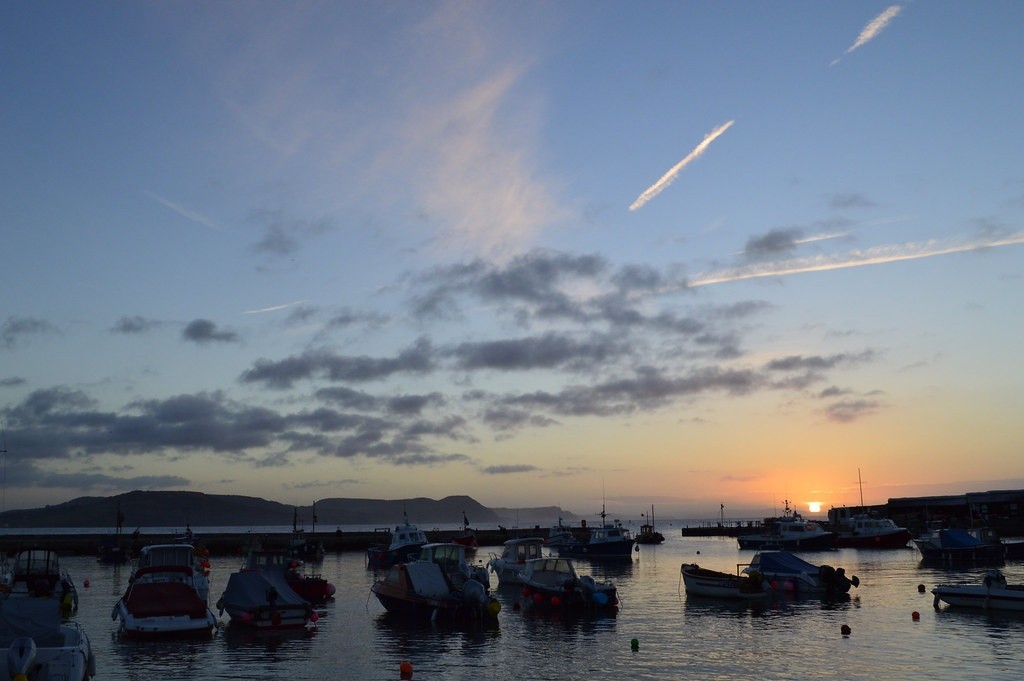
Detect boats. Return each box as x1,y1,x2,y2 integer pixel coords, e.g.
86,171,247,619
911,523,1024,572
453,507,479,546
0,545,100,681
367,514,437,566
547,518,633,560
113,535,221,649
285,512,329,570
680,547,861,612
635,505,667,547
835,506,917,552
372,542,504,639
497,534,621,631
735,513,836,552
932,570,1024,619
225,548,336,636
